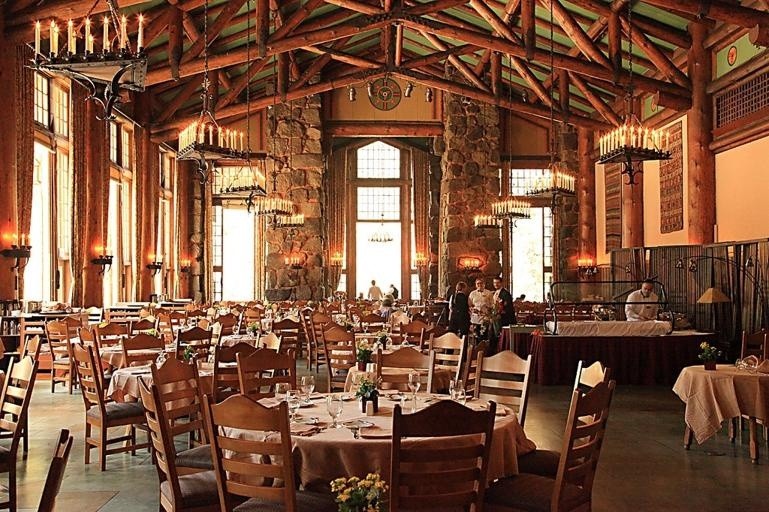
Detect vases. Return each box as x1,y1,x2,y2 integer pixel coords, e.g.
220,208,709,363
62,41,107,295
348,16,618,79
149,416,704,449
703,361,716,372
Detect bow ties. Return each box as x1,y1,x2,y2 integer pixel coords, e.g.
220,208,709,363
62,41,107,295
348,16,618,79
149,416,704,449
477,290,483,293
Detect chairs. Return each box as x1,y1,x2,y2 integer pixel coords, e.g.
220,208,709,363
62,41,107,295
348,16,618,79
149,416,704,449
739,329,769,366
1,292,616,511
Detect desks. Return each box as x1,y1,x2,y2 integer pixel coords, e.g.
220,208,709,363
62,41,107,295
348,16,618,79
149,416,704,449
530,320,715,386
675,361,769,463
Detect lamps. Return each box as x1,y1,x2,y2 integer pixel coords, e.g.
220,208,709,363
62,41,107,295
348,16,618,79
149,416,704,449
472,105,504,232
23,1,148,122
411,249,486,277
177,3,247,184
489,20,531,228
280,246,346,275
576,253,768,293
256,12,293,224
2,228,199,305
525,0,575,212
271,101,304,228
599,3,674,185
345,78,435,104
211,3,267,198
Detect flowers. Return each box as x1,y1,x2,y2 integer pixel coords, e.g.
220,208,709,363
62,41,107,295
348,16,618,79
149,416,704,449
698,342,722,362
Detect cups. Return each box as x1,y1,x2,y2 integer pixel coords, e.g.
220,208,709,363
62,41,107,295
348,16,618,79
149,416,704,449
11,309,22,317
414,301,418,306
734,359,758,375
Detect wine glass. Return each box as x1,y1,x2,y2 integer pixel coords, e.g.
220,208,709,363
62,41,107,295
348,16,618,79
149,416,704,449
245,326,253,340
273,370,473,438
154,349,168,368
232,325,239,337
358,323,410,352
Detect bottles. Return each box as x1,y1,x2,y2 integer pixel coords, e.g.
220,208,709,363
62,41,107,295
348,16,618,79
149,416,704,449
196,360,202,370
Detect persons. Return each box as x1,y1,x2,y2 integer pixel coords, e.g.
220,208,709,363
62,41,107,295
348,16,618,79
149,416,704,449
358,277,525,362
624,278,659,321
